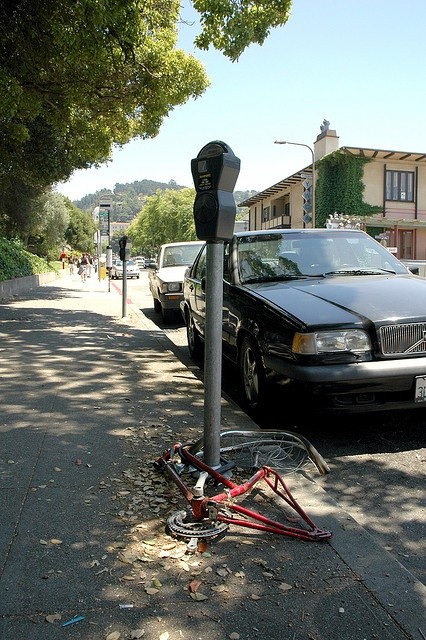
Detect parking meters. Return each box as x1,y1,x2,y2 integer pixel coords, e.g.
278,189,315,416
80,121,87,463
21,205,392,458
119,235,132,317
189,139,242,482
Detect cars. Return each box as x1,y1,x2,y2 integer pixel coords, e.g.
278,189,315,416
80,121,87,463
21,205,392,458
144,259,156,268
147,240,206,326
135,256,145,268
181,229,425,414
110,260,138,280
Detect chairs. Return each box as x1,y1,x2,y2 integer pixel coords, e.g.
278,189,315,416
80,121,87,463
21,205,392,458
278,252,299,263
227,251,262,281
167,254,181,263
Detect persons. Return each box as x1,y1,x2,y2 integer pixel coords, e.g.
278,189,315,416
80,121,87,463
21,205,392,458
68,253,75,276
78,253,92,283
86,253,98,278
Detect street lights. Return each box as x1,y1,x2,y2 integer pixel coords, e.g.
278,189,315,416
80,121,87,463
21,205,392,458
275,140,317,226
